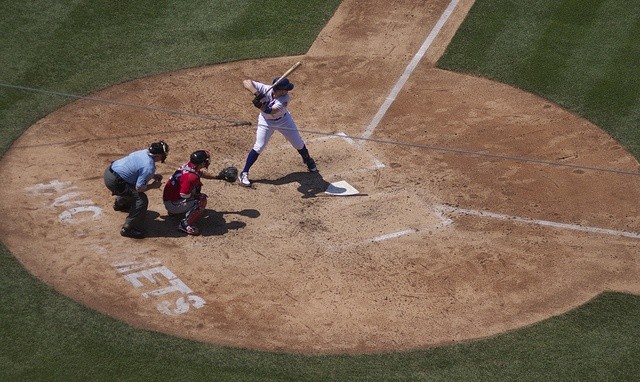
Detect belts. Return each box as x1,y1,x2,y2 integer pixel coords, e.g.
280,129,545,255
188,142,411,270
109,161,126,184
262,113,287,122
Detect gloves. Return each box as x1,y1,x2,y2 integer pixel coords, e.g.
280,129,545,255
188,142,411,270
252,98,264,109
254,91,266,100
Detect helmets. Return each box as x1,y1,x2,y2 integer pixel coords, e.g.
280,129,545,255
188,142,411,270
147,140,170,164
190,150,210,171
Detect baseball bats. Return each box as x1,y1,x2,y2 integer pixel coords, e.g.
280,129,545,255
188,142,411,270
263,61,302,95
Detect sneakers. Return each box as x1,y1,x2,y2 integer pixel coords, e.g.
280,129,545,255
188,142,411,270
238,172,251,187
178,222,200,235
307,158,317,172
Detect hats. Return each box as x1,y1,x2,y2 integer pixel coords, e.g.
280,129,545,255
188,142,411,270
273,76,294,91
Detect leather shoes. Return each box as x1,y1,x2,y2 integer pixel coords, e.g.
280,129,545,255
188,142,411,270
120,227,145,239
113,205,130,212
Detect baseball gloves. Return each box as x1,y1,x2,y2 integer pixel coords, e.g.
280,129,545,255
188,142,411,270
219,167,239,182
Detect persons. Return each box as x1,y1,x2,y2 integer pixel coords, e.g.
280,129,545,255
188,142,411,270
102,139,170,238
161,149,221,236
238,76,318,187
